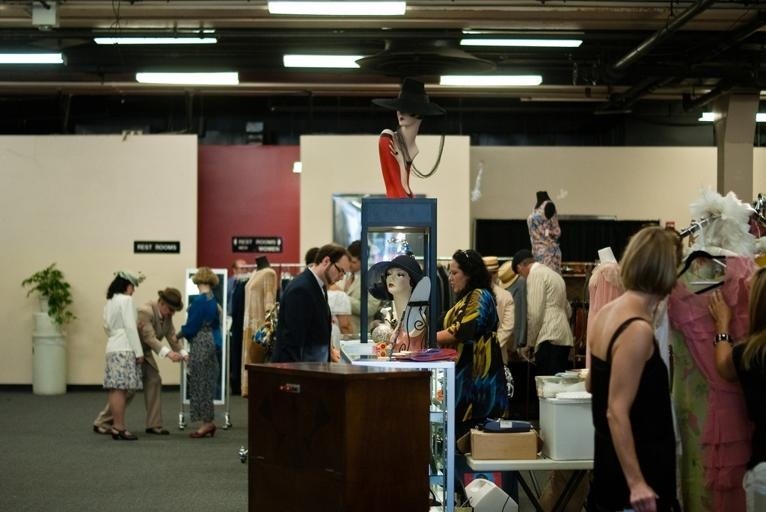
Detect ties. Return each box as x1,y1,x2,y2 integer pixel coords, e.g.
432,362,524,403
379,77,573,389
322,285,328,303
343,271,354,292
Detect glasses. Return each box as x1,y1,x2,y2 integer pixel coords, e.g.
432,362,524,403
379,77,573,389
333,261,346,275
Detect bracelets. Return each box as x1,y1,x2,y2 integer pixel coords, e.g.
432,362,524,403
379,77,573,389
712,334,733,345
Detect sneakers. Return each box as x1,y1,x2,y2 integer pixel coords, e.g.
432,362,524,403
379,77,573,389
145,426,169,435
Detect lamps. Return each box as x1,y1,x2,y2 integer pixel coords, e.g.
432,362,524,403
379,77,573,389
281,51,376,69
266,0,408,18
438,68,545,88
457,27,587,50
134,70,241,88
90,28,219,47
0,51,69,67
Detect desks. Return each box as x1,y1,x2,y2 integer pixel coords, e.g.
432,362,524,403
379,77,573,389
463,452,595,512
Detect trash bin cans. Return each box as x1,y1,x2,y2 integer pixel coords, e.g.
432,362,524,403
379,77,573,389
28,310,70,396
743,462,766,512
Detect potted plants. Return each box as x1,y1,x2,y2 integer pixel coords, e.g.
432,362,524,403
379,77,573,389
21,261,81,329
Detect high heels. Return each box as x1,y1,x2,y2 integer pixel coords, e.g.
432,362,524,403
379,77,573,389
188,424,216,438
93,424,137,440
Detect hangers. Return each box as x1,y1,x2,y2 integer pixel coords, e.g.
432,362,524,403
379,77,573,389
675,209,766,311
232,263,295,284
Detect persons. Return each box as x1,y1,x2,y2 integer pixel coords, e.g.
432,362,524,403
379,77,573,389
529,191,563,273
172,265,221,439
222,259,246,316
372,250,428,360
583,223,688,511
511,248,575,436
240,254,279,397
666,251,729,511
707,268,766,511
92,287,191,434
272,241,351,363
304,239,382,359
380,80,429,202
437,246,519,511
104,268,144,440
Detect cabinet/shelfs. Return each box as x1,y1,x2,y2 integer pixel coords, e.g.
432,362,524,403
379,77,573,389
339,339,456,512
243,361,433,512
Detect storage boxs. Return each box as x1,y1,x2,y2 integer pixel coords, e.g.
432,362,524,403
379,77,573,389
538,395,596,461
470,427,538,460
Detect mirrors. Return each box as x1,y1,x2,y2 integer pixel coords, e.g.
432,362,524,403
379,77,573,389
402,276,432,334
366,224,431,343
177,267,233,432
333,198,361,343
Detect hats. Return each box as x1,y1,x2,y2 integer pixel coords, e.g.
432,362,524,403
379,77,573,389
157,286,183,311
113,269,138,287
481,255,499,271
366,255,423,299
370,77,446,117
188,267,219,285
511,250,533,274
496,261,518,289
